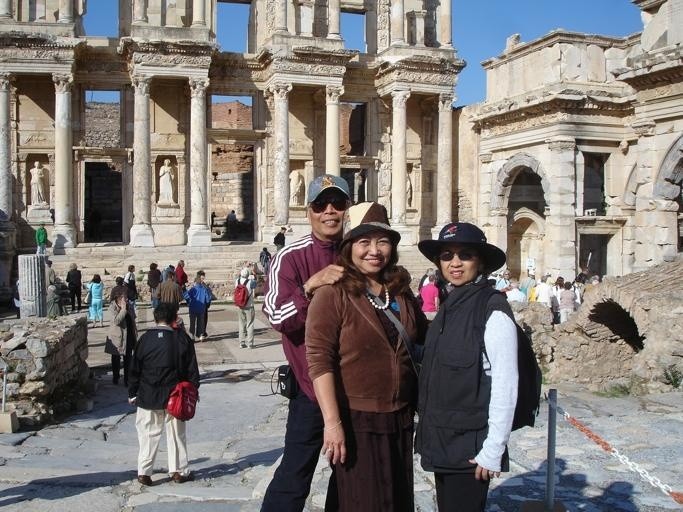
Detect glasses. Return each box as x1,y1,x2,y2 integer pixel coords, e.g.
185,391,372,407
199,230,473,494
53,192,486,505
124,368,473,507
436,251,478,261
310,200,347,214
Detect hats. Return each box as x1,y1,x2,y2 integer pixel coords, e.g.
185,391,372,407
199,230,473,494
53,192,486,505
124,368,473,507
418,222,506,275
338,202,401,244
70,263,77,269
308,174,350,204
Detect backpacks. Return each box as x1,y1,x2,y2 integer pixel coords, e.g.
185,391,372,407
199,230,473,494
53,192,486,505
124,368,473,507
234,277,250,309
474,286,541,431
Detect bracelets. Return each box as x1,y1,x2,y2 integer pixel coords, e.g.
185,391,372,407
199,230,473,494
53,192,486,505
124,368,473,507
324,420,342,434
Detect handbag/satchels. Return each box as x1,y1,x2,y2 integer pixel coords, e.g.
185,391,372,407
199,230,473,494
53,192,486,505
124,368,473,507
84,292,91,304
166,381,199,421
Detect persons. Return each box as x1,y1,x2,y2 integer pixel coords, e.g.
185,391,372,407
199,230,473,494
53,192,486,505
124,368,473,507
259,247,271,276
260,174,352,512
66,260,217,485
226,210,237,238
46,285,68,317
290,170,305,206
157,158,175,204
305,201,428,512
418,268,433,291
406,172,412,209
420,275,440,312
46,259,55,291
417,222,520,512
36,220,49,254
212,212,216,226
234,264,257,349
29,160,48,207
14,279,20,319
492,258,601,324
274,226,287,251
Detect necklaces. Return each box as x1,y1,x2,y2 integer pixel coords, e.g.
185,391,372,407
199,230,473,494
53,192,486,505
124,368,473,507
364,282,393,310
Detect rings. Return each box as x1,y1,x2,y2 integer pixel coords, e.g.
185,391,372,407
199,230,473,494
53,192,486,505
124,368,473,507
327,447,334,454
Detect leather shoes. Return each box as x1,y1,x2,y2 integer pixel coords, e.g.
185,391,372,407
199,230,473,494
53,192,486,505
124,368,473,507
137,475,153,486
174,473,194,483
